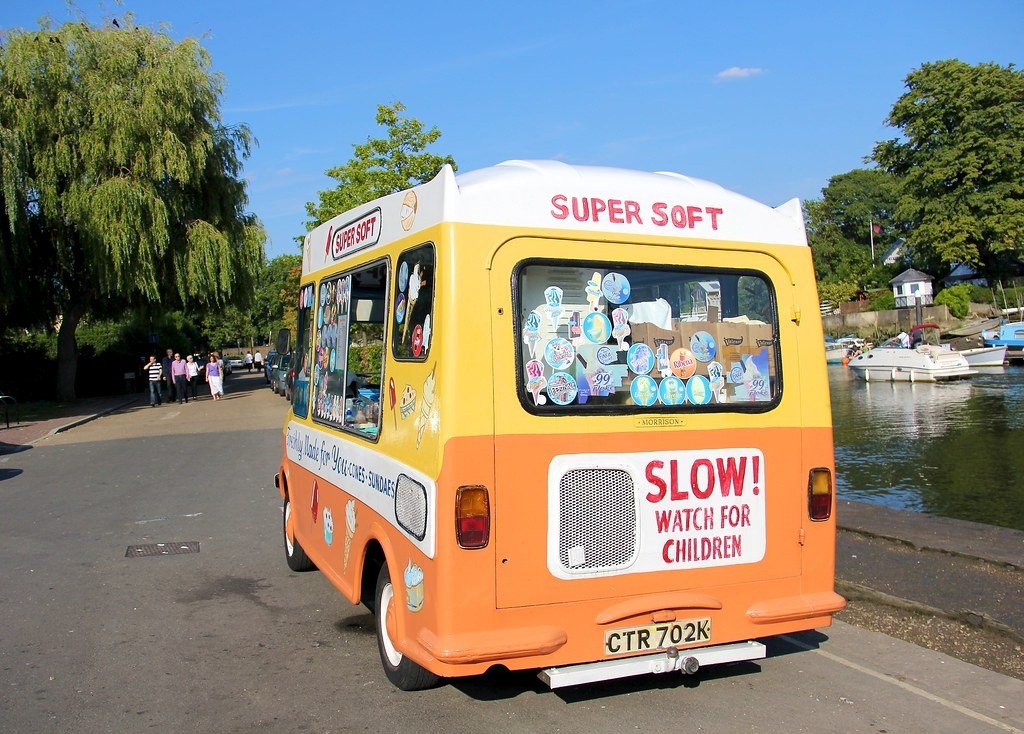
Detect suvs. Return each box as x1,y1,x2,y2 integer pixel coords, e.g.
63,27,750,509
263,349,295,405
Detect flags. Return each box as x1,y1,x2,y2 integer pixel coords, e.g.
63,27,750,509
871,223,883,234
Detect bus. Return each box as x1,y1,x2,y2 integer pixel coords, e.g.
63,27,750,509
276,159,847,691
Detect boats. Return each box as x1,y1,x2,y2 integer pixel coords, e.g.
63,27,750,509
981,318,1024,361
825,324,1009,383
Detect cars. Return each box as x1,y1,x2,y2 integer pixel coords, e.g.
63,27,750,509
227,356,244,369
195,358,234,383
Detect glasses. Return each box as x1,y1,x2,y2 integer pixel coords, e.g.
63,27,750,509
175,356,180,358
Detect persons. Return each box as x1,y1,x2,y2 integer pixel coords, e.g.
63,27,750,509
143,349,263,406
852,325,923,359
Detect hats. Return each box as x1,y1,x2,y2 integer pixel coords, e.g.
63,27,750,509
213,352,220,357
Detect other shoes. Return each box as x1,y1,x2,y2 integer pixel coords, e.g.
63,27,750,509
216,395,220,400
158,402,161,405
193,396,197,400
151,402,155,407
185,398,188,403
179,400,182,404
